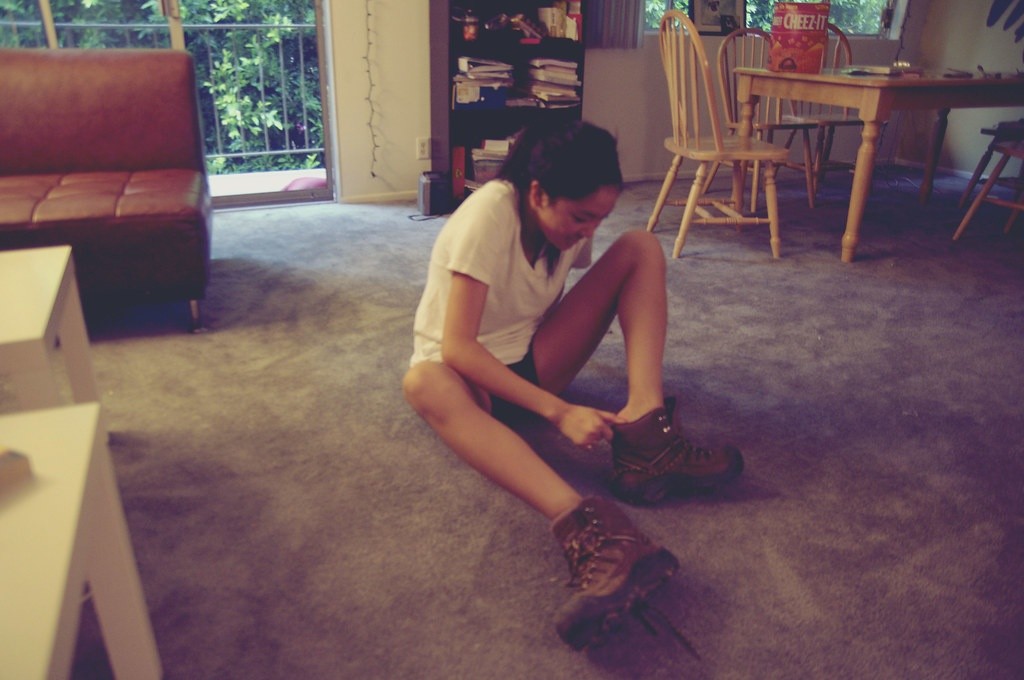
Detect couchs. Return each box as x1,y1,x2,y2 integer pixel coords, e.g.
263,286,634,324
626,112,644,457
0,48,213,333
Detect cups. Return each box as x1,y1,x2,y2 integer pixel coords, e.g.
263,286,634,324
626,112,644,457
538,7,558,37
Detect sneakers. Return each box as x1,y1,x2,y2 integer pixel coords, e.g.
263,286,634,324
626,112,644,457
550,496,704,663
610,397,745,507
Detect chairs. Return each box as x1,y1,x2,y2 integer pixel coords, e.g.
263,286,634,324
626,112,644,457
645,10,789,259
949,116,1023,240
773,22,888,195
716,28,819,209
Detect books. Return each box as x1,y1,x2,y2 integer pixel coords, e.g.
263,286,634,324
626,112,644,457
847,65,924,74
452,56,582,163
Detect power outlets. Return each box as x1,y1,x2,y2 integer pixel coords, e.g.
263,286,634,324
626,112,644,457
416,134,432,161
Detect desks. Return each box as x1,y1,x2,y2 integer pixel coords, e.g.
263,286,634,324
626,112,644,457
733,65,1024,262
0,244,110,442
0,402,162,680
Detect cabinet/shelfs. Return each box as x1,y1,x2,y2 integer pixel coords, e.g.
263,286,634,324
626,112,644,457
430,0,584,208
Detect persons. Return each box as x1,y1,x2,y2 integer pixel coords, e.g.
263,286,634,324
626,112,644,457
404,123,744,647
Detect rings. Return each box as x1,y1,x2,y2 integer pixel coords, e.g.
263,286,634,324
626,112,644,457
599,438,607,448
588,445,592,448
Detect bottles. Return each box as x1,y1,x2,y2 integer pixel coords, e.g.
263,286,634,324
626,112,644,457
463,9,480,41
